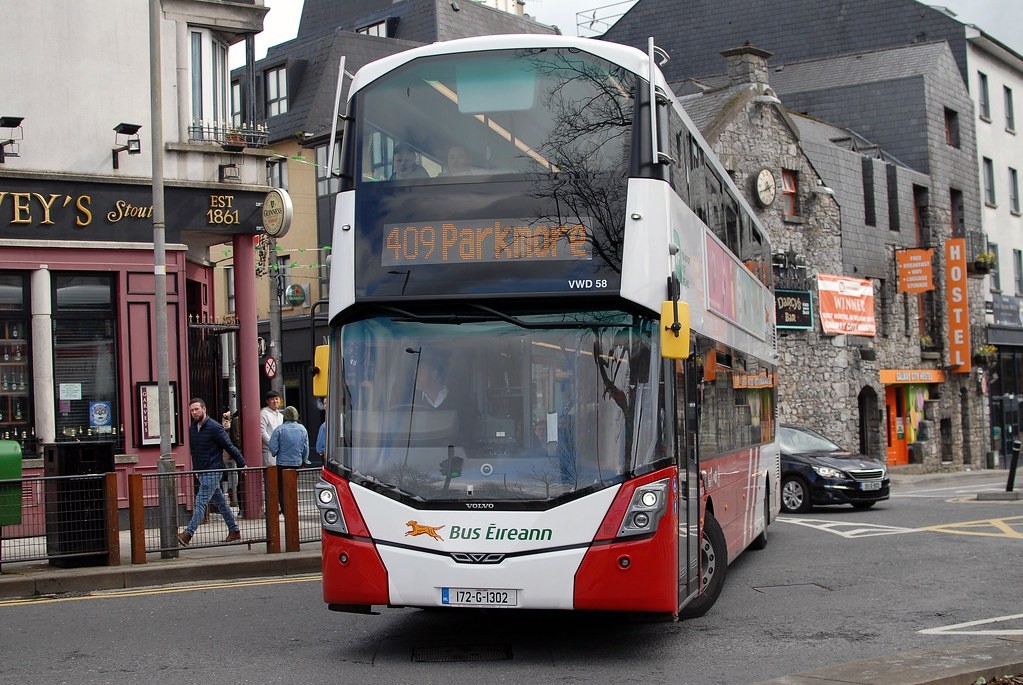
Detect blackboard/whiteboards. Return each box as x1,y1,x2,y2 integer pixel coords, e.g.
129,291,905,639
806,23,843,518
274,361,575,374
775,290,814,329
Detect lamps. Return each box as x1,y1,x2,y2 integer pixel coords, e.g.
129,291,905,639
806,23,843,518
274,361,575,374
219,164,242,184
0,117,25,163
112,122,142,169
810,180,835,195
752,96,781,105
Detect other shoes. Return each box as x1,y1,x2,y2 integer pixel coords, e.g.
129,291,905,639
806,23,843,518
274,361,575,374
220,530,241,543
178,531,191,547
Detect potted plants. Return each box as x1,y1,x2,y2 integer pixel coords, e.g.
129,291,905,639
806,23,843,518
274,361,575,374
975,252,995,274
920,335,934,351
973,345,998,367
225,128,247,146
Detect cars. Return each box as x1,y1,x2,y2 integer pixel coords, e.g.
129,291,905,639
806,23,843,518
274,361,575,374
779,422,891,515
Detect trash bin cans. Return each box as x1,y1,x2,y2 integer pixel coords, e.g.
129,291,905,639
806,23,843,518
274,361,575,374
41,441,117,569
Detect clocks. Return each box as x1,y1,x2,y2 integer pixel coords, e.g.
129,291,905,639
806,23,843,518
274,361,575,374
754,167,776,209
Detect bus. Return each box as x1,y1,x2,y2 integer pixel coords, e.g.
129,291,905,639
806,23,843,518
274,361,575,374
306,29,784,627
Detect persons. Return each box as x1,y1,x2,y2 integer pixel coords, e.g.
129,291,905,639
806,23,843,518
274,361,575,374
178,398,241,546
269,406,309,521
390,144,416,180
261,391,283,466
315,421,326,466
412,356,481,417
221,411,242,518
447,145,469,175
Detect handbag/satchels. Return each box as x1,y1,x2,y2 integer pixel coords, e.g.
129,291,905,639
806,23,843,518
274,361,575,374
221,464,228,481
